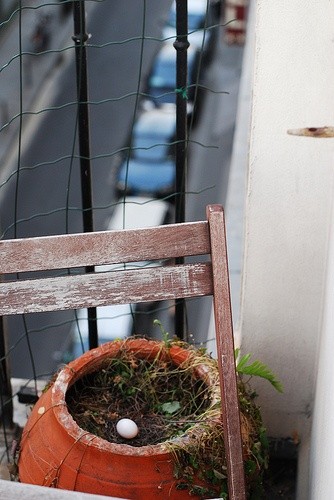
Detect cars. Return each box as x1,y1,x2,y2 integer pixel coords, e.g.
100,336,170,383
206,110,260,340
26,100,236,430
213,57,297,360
163,0,215,30
51,194,173,369
142,26,213,105
111,97,196,202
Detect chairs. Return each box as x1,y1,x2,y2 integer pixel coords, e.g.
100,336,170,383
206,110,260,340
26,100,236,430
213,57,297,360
0,202,250,499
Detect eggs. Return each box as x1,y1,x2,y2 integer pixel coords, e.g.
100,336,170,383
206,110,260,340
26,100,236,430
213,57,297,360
117,418,139,438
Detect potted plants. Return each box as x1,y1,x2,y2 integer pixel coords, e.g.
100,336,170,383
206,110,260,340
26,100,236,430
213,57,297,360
15,318,285,500
